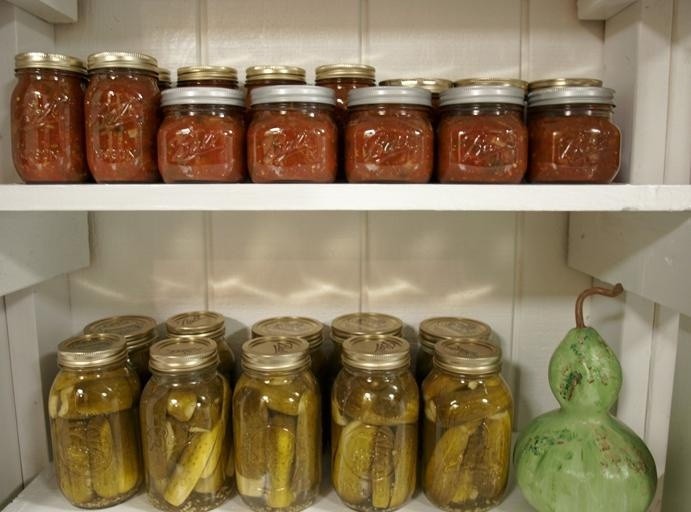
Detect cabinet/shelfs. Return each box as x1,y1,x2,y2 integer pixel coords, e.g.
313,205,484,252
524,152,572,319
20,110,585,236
0,0,691,512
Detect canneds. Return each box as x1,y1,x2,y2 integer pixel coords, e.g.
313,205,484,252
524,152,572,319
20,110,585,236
49,311,514,512
10,52,621,183
